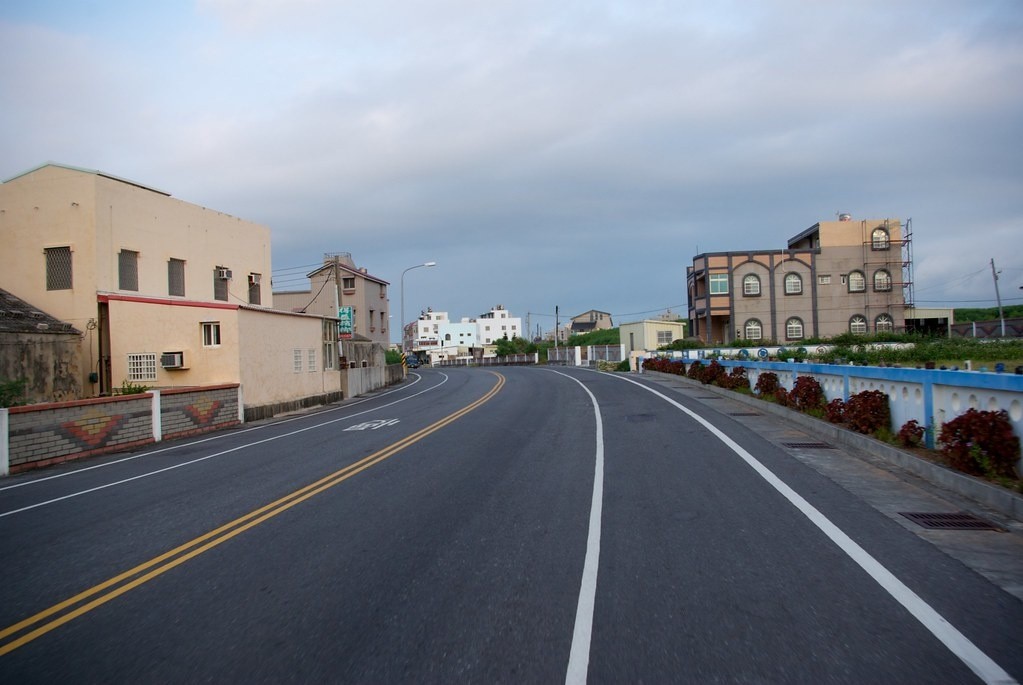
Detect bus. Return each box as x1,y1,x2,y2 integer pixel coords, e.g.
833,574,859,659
404,355,419,369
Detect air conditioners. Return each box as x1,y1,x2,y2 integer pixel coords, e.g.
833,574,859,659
218,269,231,280
248,275,259,283
159,354,182,368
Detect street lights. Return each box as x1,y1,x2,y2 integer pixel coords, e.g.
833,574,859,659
400,261,436,380
